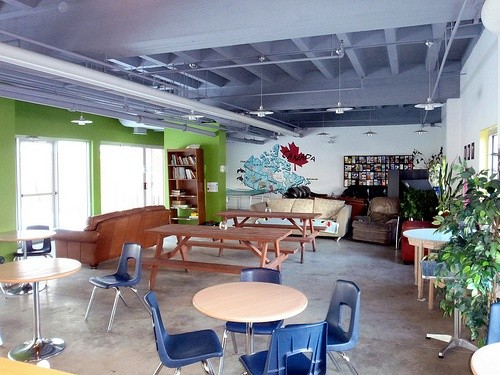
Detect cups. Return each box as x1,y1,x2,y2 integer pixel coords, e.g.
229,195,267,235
220,220,228,231
265,207,272,213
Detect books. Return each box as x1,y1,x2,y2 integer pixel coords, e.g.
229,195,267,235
172,155,196,179
171,189,186,195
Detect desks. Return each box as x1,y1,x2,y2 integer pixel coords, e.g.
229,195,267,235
193,282,308,375
470,342,500,375
0,256,81,362
0,229,56,295
146,223,292,292
419,260,479,358
214,209,323,265
404,229,479,299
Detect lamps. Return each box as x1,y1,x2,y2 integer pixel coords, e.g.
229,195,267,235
414,117,427,135
70,53,353,137
414,44,443,110
362,112,376,136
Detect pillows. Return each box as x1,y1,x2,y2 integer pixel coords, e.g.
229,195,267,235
313,197,345,219
292,200,313,213
266,199,292,212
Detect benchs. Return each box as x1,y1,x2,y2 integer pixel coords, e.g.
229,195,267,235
129,226,327,276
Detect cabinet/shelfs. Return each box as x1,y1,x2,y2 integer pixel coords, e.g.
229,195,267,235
343,155,414,187
166,148,206,224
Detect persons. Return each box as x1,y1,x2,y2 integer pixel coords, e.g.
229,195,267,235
343,155,413,186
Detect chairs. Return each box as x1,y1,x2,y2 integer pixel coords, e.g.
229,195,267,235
0,225,361,375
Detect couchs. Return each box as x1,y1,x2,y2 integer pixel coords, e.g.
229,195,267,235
52,204,170,269
352,197,399,243
401,213,449,262
251,202,351,233
